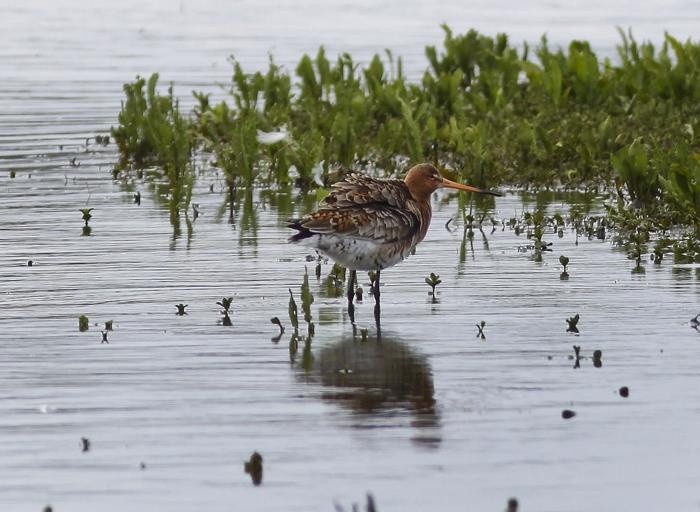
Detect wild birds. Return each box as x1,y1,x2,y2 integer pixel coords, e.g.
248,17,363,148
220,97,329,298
284,160,504,306
